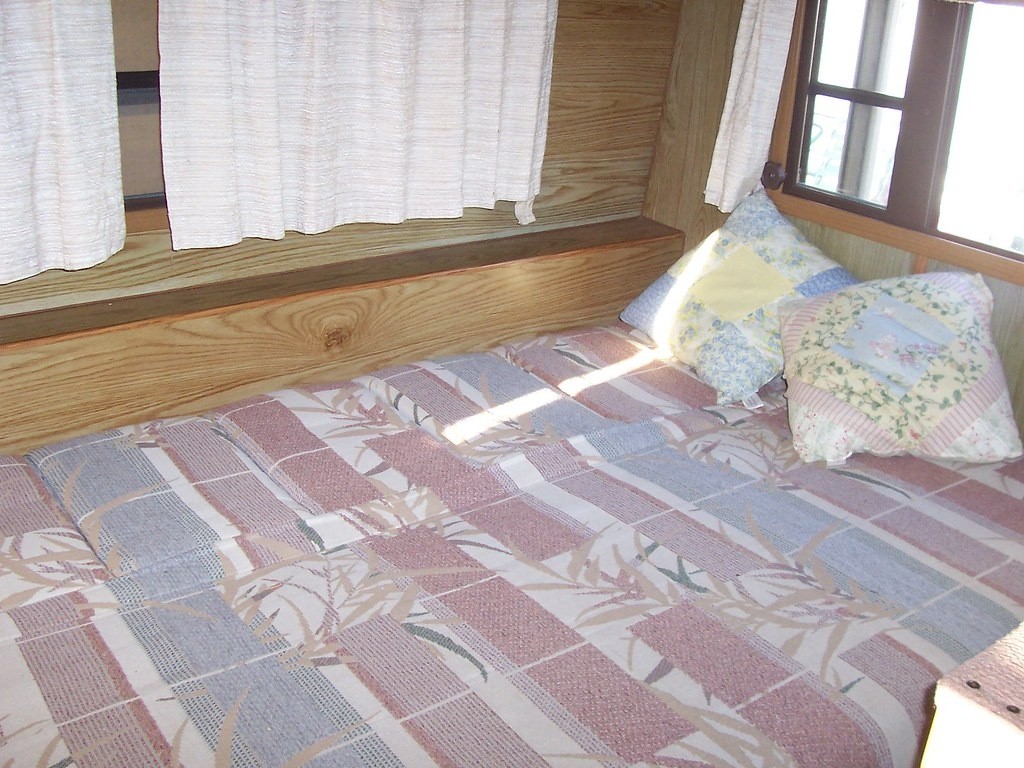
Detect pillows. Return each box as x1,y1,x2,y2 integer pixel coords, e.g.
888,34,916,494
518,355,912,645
621,182,861,402
777,273,1024,465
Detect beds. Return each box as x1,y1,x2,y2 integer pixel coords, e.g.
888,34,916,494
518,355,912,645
1,184,1023,768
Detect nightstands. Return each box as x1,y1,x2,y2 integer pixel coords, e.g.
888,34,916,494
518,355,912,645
918,622,1022,768
